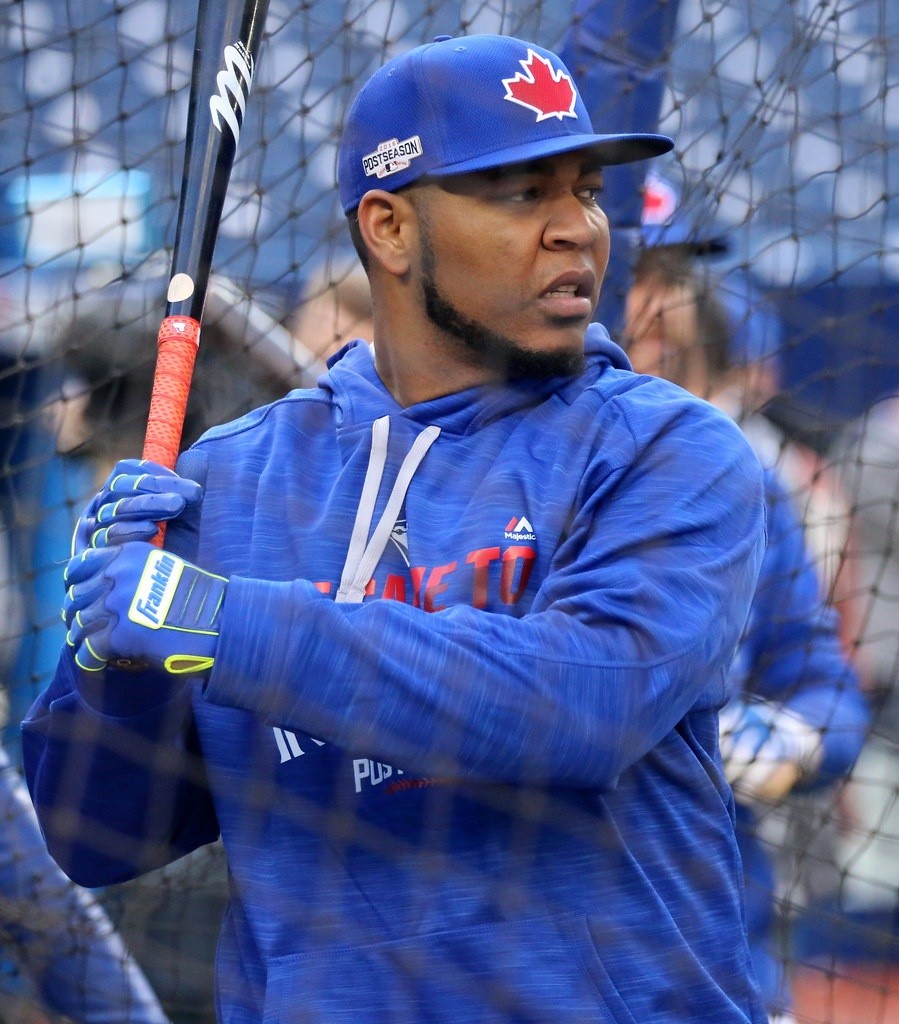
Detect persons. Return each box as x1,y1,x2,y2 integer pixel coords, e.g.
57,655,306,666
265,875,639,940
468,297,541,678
0,33,872,1024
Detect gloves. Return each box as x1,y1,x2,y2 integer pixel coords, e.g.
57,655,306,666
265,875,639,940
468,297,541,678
71,459,203,552
63,541,229,676
719,698,823,805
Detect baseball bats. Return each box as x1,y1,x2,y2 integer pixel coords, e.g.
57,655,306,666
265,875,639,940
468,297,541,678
103,0,273,677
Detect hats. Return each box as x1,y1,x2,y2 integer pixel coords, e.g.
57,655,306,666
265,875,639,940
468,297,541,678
338,34,674,216
704,273,786,386
633,169,730,250
